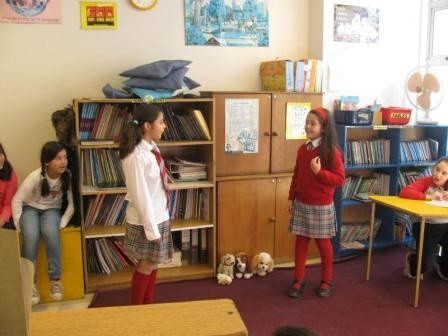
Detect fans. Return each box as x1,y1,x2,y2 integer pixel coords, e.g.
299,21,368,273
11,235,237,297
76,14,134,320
403,66,445,125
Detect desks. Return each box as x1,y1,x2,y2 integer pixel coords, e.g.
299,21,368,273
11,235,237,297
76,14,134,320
28,299,248,336
366,196,448,308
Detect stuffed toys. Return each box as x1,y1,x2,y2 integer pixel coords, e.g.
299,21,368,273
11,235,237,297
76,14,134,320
249,252,274,277
234,252,253,280
216,253,236,285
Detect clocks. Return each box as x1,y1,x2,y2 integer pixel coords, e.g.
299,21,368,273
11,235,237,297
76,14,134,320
128,0,157,11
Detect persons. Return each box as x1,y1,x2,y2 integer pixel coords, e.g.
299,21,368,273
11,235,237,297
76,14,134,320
398,155,448,280
10,141,75,306
287,107,346,298
0,142,19,230
118,104,174,305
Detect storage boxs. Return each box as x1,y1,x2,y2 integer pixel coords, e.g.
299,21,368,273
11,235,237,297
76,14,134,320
260,59,322,93
381,107,412,125
334,110,373,125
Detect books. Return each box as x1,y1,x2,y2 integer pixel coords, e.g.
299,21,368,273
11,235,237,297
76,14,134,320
340,138,439,249
79,104,213,275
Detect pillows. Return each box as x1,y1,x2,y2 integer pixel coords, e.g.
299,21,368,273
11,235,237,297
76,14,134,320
102,59,201,99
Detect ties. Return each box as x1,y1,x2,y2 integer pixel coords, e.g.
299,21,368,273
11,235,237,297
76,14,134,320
152,149,169,207
307,142,312,151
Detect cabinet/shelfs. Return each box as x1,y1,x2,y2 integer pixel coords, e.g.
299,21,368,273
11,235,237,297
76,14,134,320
199,91,324,268
333,124,448,257
24,225,85,303
73,98,216,294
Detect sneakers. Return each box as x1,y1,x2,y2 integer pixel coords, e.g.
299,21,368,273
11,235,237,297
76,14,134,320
48,280,62,299
31,283,40,305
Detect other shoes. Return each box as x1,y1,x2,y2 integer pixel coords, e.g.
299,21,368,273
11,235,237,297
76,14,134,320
289,281,304,297
406,253,416,279
432,255,441,279
317,280,332,296
